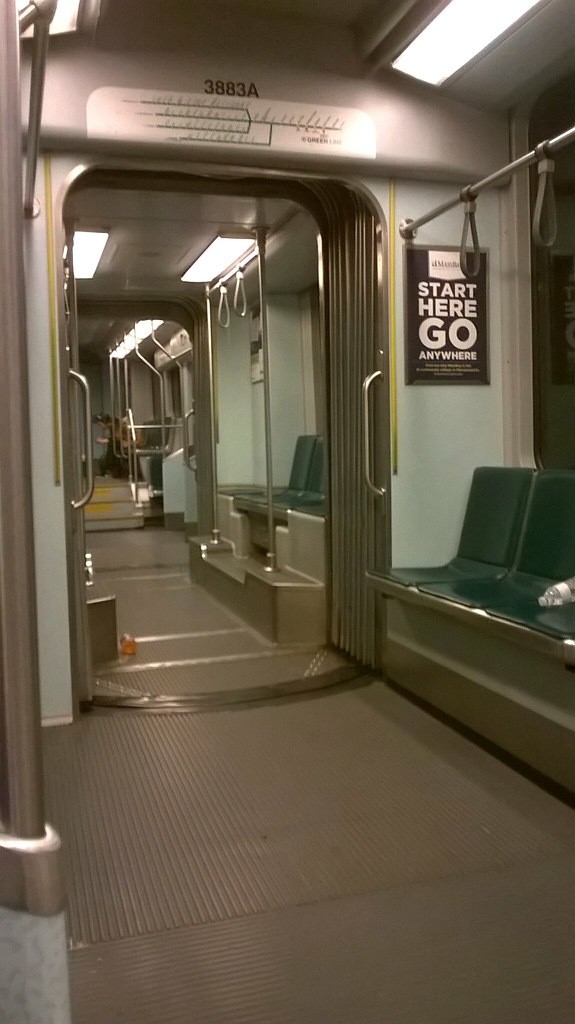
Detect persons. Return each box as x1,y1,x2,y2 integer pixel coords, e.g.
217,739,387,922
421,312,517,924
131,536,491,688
92,412,144,479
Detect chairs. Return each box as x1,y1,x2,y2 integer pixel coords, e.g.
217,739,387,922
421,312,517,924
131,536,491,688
129,416,173,450
484,599,575,641
416,468,575,611
269,436,326,511
365,466,537,588
295,504,327,520
236,434,317,506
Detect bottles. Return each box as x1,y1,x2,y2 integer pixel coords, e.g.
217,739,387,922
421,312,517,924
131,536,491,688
537,575,575,607
119,633,138,654
